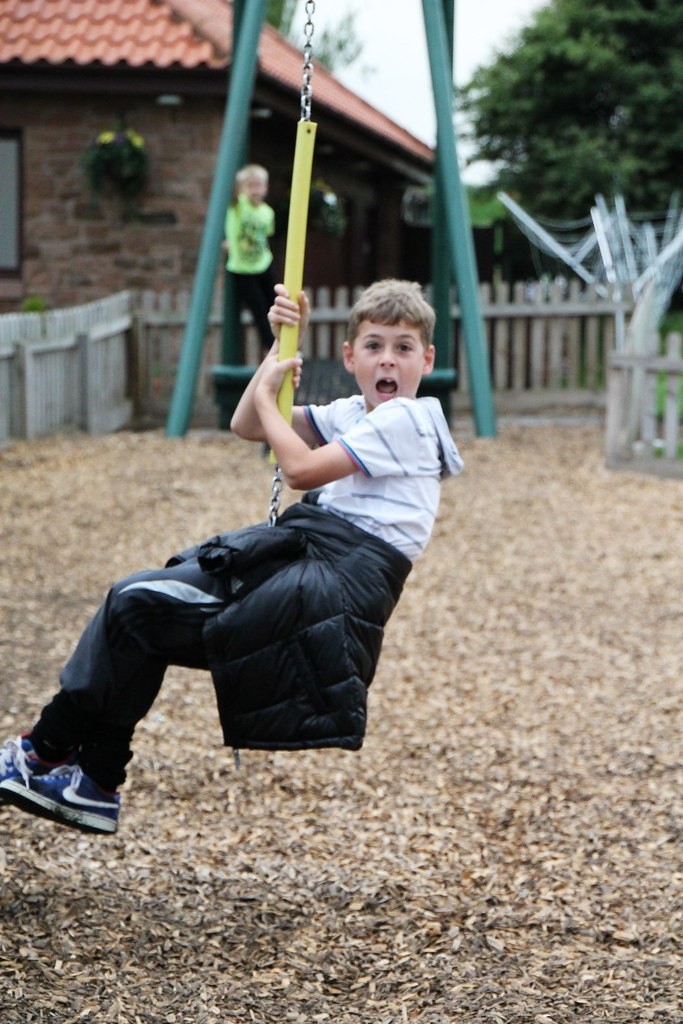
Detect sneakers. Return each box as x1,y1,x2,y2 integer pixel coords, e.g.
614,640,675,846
0,764,121,835
0,730,73,783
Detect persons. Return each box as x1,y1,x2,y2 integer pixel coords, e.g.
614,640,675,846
0,276,463,837
220,162,281,367
